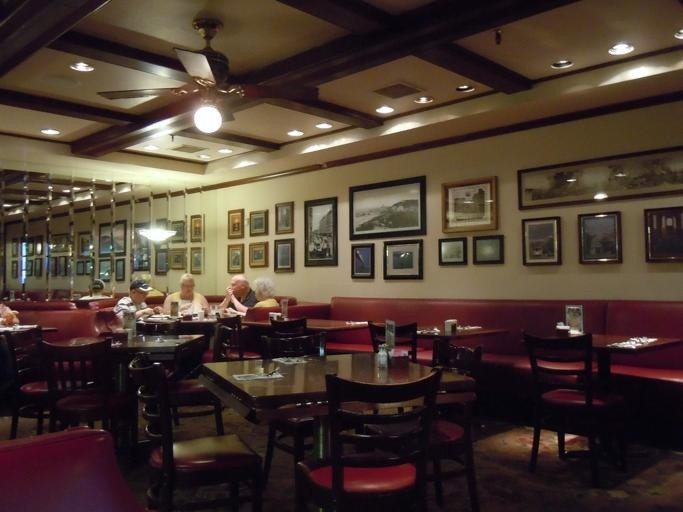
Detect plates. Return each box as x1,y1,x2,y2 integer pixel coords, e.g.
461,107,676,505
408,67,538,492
149,314,170,319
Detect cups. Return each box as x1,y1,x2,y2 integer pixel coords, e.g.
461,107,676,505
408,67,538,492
182,311,192,321
198,311,205,320
446,319,457,331
269,313,281,321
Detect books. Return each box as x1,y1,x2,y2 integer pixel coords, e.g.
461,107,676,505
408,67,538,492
274,357,306,366
233,371,285,382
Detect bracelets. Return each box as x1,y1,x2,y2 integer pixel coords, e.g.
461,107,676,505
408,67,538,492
142,309,144,314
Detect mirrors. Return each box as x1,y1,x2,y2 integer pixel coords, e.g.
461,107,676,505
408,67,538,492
0,158,217,298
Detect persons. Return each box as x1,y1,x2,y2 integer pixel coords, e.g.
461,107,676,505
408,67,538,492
0,304,20,325
78,272,162,320
163,275,210,316
217,275,257,313
309,236,330,257
253,277,280,307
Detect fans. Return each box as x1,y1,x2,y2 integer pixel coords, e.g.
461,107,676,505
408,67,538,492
95,17,320,127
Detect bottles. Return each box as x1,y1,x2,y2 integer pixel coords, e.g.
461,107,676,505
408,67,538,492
20,283,26,301
375,344,387,369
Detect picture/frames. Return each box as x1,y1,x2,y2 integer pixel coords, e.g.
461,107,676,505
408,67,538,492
3,212,206,282
437,143,682,268
223,176,426,280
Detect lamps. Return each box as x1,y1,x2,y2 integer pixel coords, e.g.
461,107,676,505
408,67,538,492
193,96,221,133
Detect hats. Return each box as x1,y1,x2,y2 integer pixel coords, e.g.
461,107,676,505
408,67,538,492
130,280,153,292
